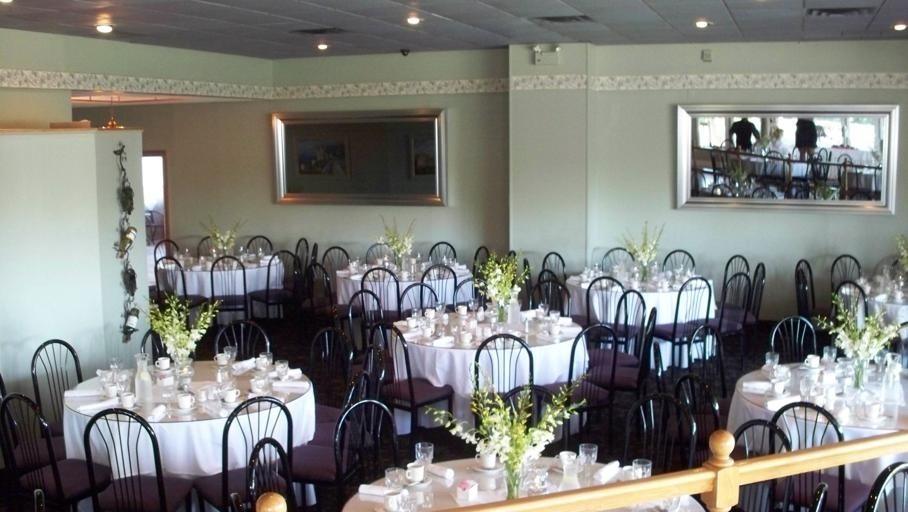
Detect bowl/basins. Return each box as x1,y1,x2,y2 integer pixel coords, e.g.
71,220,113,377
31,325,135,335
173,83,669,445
559,317,572,326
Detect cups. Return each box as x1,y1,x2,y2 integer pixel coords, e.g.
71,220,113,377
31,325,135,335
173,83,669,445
96,369,113,380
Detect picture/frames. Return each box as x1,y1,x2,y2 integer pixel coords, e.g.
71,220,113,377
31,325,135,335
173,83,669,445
270,108,447,207
674,102,900,215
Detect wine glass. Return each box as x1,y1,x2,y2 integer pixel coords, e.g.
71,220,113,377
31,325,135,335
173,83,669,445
412,298,561,344
594,262,693,289
384,443,680,512
109,347,287,414
177,245,265,270
766,347,905,416
348,250,456,281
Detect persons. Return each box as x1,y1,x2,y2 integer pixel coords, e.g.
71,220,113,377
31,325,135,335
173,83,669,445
795,118,818,161
726,117,764,154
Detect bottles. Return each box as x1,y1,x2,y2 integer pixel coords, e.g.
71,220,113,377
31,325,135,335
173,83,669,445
124,269,136,296
120,186,133,215
118,226,137,258
122,308,140,343
